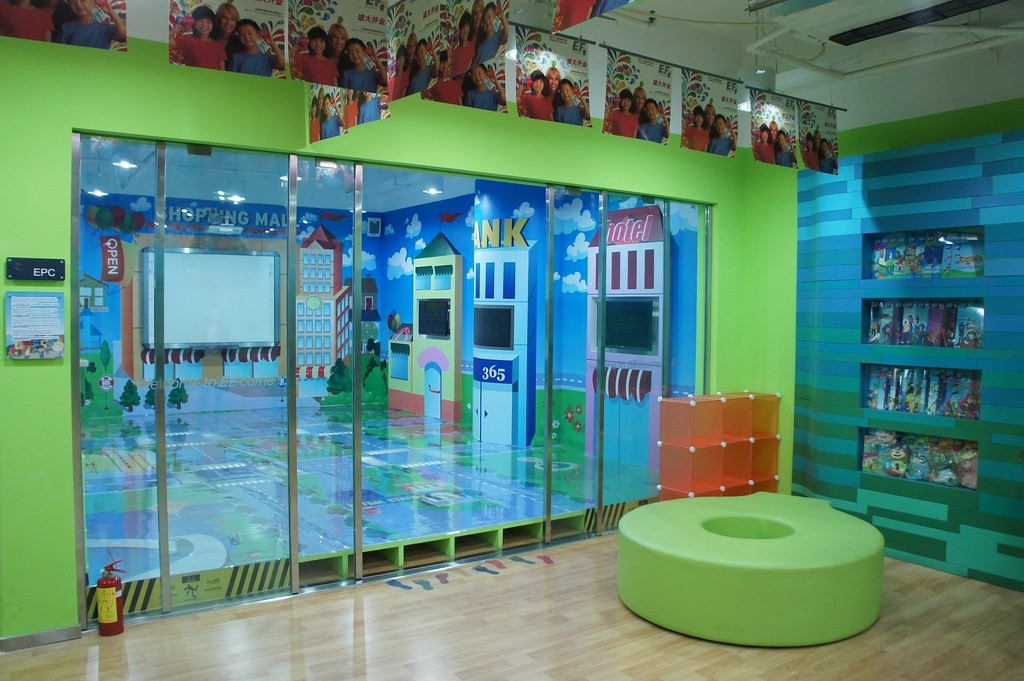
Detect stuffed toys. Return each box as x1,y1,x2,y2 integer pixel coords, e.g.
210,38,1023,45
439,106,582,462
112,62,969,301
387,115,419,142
868,429,978,491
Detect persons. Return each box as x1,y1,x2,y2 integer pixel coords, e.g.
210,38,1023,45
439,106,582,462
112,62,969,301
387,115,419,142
1,1,840,176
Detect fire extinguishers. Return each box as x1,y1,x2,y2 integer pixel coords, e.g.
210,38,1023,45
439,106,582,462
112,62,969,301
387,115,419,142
97,559,127,636
